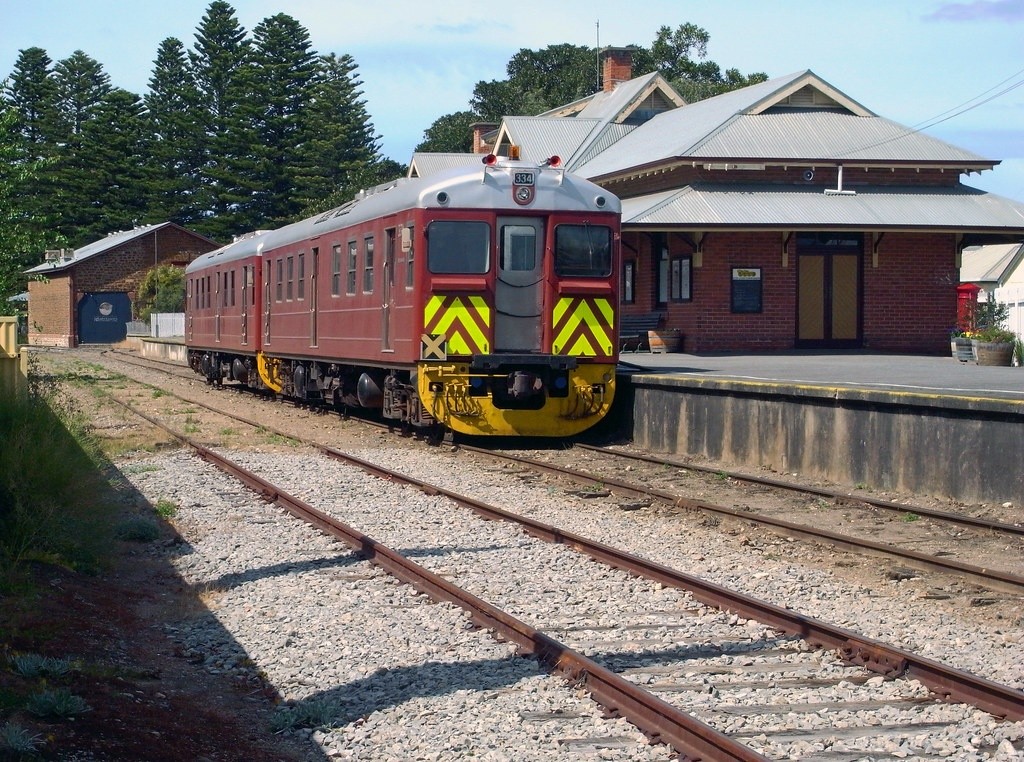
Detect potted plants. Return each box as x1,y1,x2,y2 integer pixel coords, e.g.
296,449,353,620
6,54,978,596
970,324,1016,366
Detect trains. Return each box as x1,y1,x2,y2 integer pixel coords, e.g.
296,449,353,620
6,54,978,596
183,143,620,442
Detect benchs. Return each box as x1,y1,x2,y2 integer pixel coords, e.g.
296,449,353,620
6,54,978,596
620,313,661,354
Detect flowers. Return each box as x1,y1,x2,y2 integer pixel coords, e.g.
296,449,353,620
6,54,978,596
946,325,982,339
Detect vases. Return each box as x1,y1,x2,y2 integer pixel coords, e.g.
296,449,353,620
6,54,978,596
950,336,973,365
647,328,682,354
